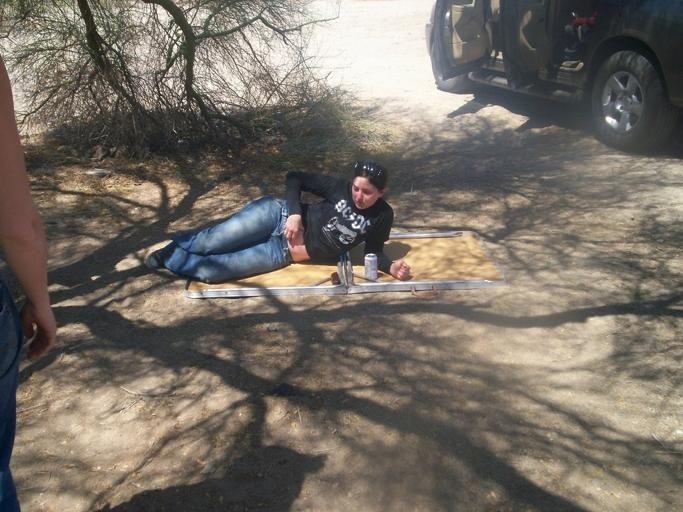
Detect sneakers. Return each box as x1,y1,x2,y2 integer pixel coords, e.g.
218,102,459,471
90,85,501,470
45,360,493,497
146,242,177,268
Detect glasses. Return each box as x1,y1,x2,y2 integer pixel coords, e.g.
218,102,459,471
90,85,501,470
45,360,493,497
354,160,384,177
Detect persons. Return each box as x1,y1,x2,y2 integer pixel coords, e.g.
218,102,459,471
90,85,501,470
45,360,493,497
0,55,58,511
142,158,411,285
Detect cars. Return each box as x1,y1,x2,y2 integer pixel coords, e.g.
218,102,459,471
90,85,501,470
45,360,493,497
425,0,680,154
